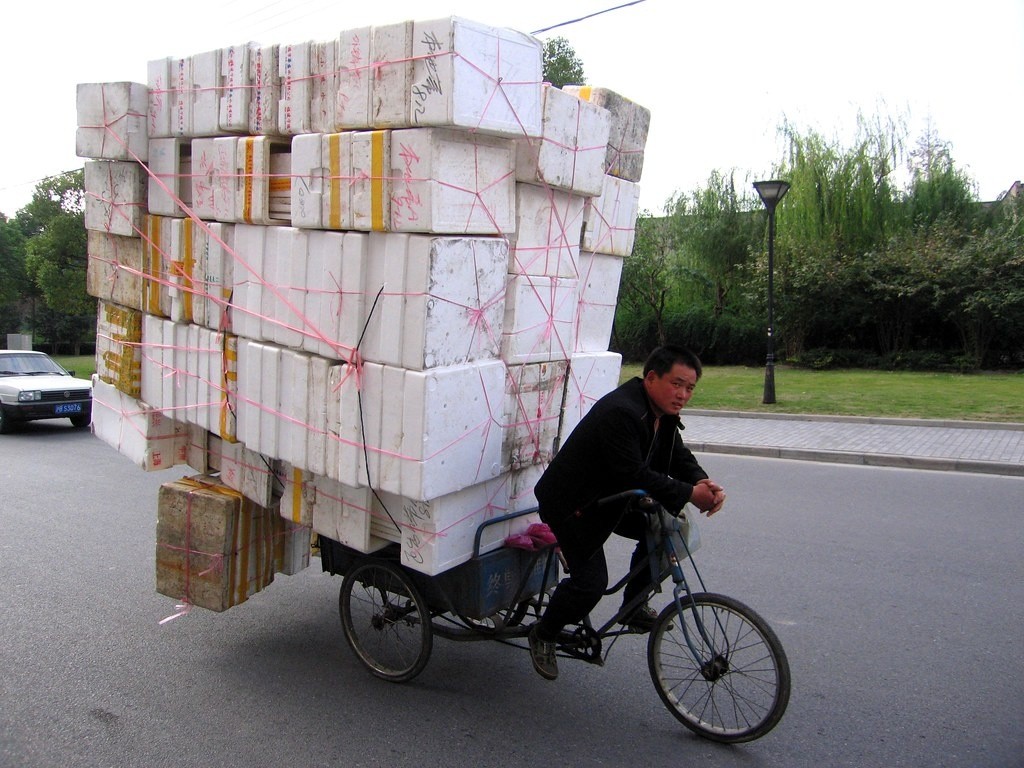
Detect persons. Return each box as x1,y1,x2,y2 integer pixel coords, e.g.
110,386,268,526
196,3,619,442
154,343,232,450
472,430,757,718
528,345,725,684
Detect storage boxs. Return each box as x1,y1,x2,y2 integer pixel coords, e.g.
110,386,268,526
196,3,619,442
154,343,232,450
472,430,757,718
77,13,650,610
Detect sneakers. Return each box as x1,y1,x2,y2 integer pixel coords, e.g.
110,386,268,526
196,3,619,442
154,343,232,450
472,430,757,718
617,602,674,631
528,626,559,681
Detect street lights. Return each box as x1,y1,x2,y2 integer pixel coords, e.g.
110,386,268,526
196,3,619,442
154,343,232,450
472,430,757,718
753,180,791,404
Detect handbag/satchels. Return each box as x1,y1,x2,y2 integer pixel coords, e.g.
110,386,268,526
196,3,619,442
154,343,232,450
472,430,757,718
639,492,701,580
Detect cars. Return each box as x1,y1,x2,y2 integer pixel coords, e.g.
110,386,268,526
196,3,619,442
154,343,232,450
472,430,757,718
0,350,92,434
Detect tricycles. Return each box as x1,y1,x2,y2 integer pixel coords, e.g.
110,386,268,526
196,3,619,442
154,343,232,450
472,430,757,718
311,489,791,744
